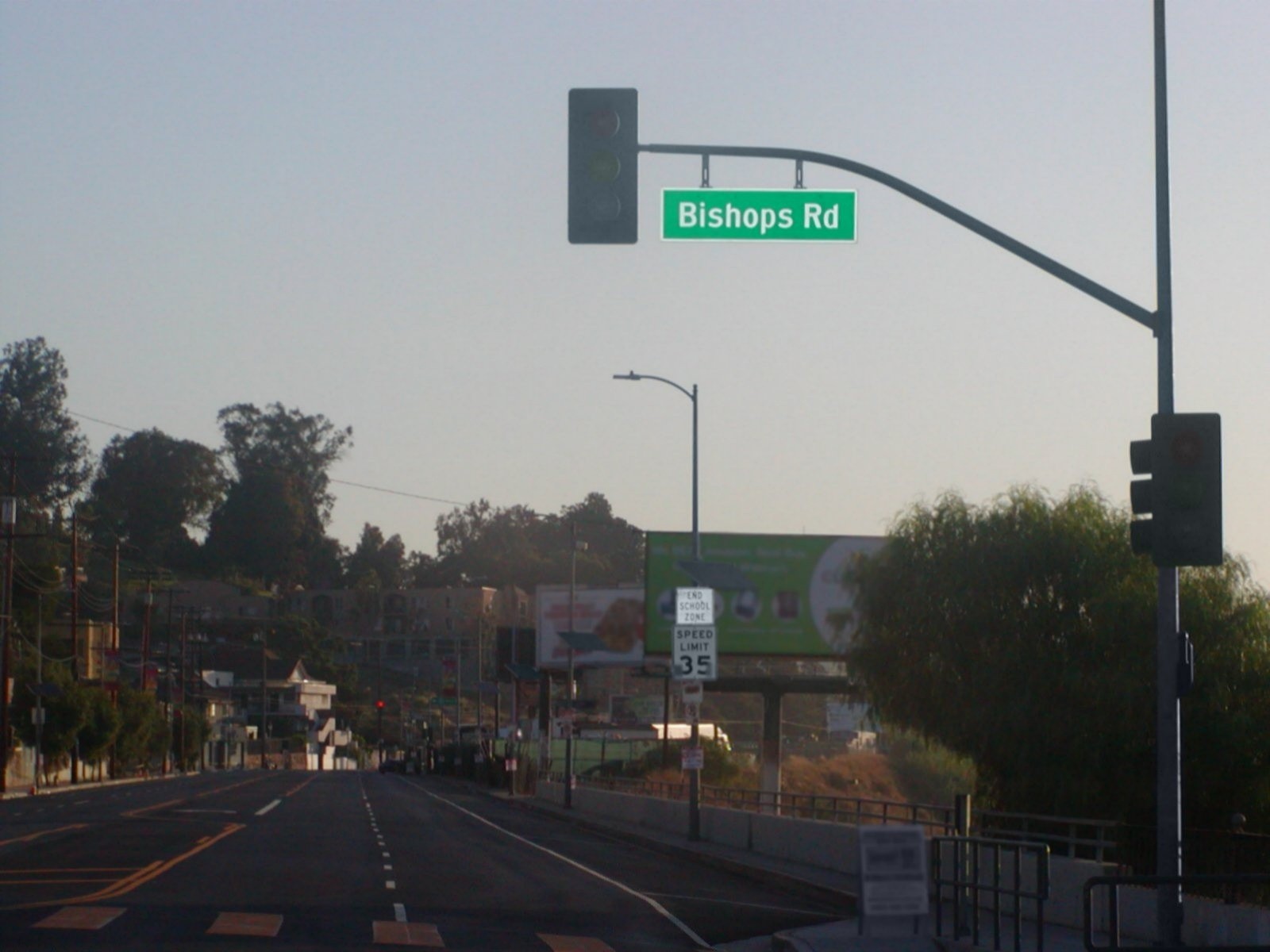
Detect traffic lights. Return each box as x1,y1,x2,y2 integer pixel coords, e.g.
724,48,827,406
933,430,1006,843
562,85,643,243
1127,411,1229,569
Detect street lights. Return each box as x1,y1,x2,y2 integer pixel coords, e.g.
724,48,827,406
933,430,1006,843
613,368,710,862
